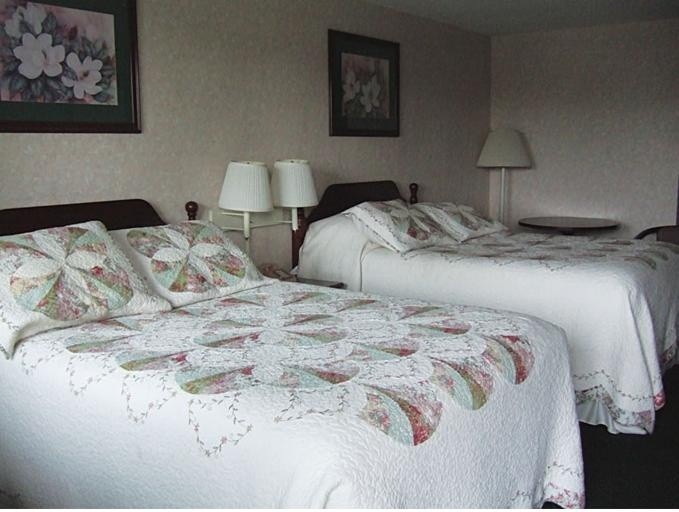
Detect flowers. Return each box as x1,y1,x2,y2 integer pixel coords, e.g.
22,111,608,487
1,3,114,103
338,63,383,118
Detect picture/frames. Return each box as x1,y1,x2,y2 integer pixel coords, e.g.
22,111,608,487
0,1,142,135
328,27,402,138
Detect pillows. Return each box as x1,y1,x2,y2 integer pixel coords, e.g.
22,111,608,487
350,200,509,255
0,218,277,358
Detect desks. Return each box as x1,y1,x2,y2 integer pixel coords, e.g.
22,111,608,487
518,215,620,235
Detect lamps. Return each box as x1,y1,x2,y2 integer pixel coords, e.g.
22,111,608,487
476,128,532,226
209,158,319,241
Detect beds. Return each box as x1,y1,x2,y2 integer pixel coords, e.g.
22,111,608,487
1,200,575,509
297,171,678,434
